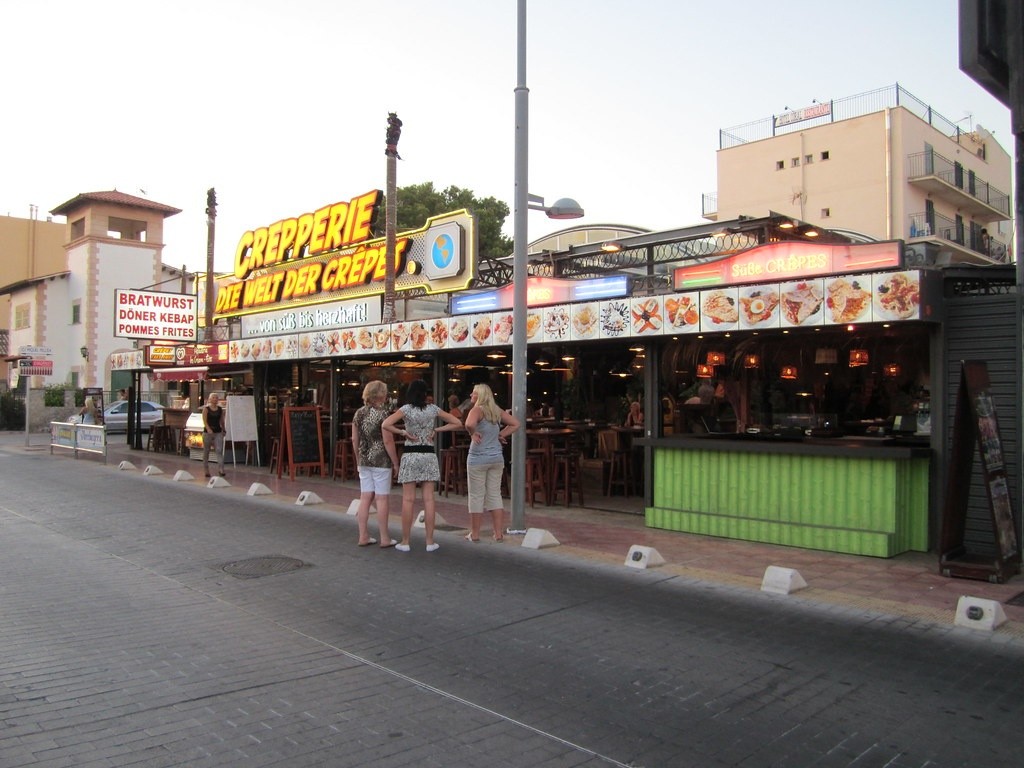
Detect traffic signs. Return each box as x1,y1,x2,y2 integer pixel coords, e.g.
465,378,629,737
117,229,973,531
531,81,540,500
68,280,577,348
15,347,57,379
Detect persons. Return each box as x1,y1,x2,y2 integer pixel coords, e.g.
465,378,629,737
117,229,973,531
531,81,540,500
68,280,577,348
446,394,464,426
203,393,226,478
381,379,465,551
624,402,645,429
79,396,101,425
239,381,252,395
684,384,738,433
464,384,521,543
352,379,400,548
118,388,128,400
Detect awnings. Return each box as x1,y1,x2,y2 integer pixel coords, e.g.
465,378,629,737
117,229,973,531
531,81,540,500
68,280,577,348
153,364,252,382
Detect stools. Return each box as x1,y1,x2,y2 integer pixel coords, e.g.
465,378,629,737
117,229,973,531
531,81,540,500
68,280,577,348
147,423,643,509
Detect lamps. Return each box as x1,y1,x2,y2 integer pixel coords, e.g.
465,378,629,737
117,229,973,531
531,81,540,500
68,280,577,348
628,342,645,352
742,354,762,368
600,241,625,253
784,104,793,112
778,219,793,230
347,376,361,387
81,345,90,362
882,361,901,377
697,364,714,378
796,345,814,397
486,342,576,383
812,99,822,105
800,226,820,237
711,227,731,238
848,344,872,367
631,357,645,370
780,363,799,380
706,351,727,366
449,366,464,382
611,365,633,377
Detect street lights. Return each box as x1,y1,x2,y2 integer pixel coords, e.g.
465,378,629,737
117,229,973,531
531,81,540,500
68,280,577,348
497,0,583,531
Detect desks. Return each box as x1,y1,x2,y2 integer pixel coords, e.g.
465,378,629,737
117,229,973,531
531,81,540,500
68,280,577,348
598,427,646,495
568,423,613,459
526,429,577,501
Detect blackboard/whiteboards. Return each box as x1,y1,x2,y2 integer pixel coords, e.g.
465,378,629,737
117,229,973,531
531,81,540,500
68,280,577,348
278,406,325,468
223,395,259,442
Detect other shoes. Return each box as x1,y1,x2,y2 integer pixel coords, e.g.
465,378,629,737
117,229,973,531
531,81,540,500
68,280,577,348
219,470,226,476
426,542,440,552
395,544,410,552
205,473,210,477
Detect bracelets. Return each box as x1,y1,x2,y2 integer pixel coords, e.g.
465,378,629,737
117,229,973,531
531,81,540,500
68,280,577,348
223,431,226,433
434,430,437,433
401,429,407,437
469,431,474,435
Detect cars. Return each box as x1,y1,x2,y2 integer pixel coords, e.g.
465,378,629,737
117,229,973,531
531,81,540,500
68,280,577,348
65,400,169,433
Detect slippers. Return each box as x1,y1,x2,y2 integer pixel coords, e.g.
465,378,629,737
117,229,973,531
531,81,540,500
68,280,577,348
379,537,398,548
464,532,480,543
358,537,376,547
493,534,504,543
